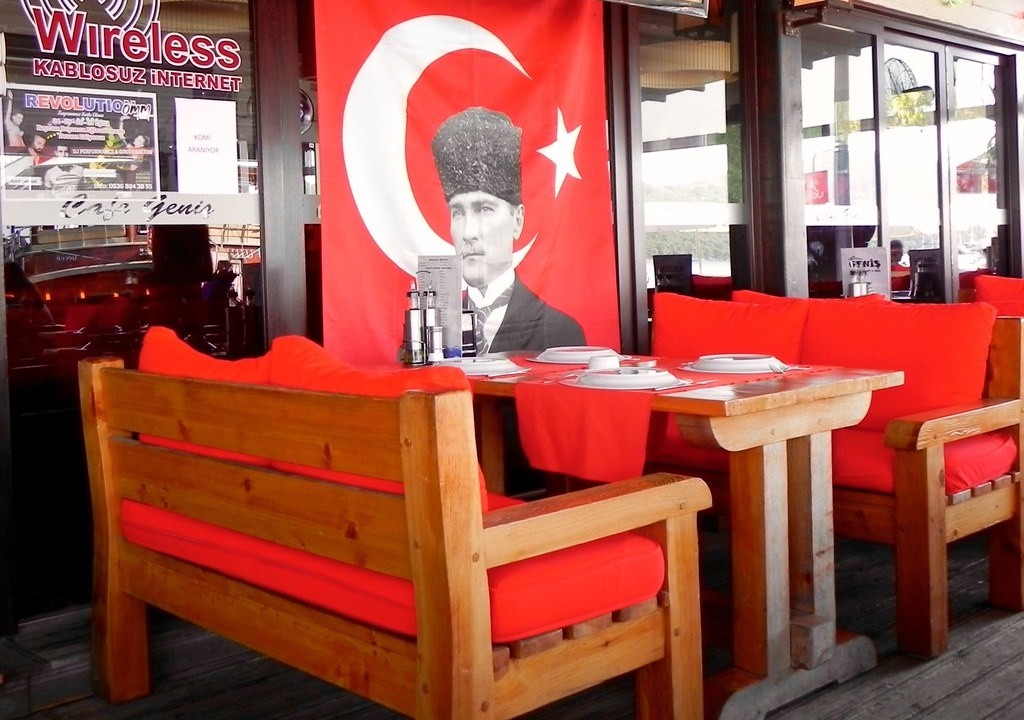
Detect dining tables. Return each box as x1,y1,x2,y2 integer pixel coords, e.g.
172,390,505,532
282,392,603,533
414,333,905,720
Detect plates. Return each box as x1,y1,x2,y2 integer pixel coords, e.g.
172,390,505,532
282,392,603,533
542,346,614,362
442,355,513,373
578,368,676,386
693,354,778,372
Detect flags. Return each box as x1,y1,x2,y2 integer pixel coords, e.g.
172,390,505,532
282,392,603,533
314,0,622,370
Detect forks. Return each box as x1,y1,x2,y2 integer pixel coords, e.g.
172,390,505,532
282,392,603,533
769,363,813,374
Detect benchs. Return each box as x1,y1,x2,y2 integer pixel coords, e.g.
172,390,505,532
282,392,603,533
79,340,715,720
603,297,1022,655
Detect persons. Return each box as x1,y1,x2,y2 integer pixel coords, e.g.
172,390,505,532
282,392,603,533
45,144,87,191
890,239,911,292
113,116,151,184
431,107,585,353
11,134,46,178
5,88,25,148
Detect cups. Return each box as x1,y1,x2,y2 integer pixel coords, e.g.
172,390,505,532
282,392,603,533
589,355,622,372
